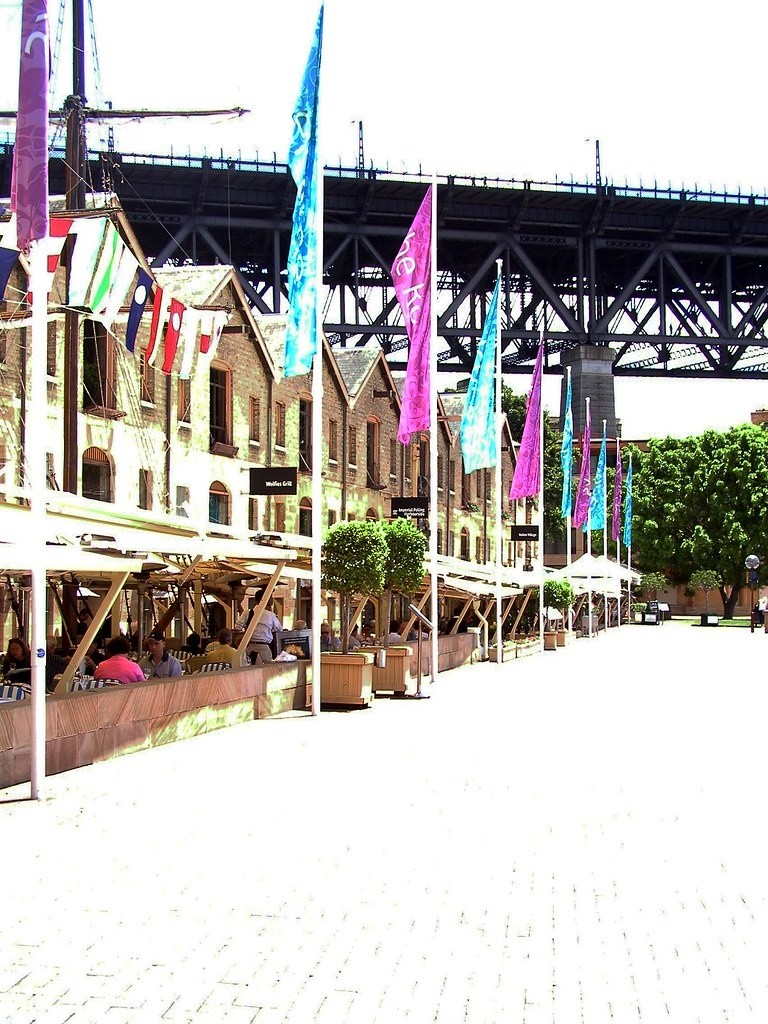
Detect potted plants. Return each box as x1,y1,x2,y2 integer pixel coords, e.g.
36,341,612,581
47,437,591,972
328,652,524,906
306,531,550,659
631,602,646,622
688,569,723,626
536,579,572,650
321,516,428,708
641,572,665,623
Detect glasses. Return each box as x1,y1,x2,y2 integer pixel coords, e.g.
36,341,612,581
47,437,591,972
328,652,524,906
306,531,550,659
321,631,329,635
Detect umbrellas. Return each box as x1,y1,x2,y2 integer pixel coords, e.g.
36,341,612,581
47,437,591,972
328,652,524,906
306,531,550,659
554,554,642,579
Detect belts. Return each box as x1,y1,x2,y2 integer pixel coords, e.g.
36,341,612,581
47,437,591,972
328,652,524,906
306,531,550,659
250,641,266,644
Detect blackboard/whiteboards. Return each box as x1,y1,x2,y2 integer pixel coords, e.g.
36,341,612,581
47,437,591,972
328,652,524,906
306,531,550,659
391,497,428,517
249,469,298,496
511,526,539,541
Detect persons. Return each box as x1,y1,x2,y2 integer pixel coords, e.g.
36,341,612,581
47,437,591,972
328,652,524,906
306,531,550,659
138,631,182,679
445,607,495,640
235,590,283,663
389,620,430,641
205,636,220,651
320,623,339,652
206,628,237,667
348,622,371,648
758,592,767,629
94,636,145,684
294,620,307,630
181,634,205,656
3,608,107,685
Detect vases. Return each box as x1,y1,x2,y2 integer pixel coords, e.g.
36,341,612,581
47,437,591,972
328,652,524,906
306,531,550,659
489,641,541,662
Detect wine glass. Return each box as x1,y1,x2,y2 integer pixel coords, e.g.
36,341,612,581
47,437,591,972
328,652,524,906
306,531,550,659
144,668,151,681
79,677,89,690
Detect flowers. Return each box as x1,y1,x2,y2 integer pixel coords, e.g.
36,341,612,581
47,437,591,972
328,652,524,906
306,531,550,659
491,636,539,647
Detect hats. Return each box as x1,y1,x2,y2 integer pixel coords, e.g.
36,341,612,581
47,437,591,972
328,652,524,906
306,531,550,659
147,632,164,642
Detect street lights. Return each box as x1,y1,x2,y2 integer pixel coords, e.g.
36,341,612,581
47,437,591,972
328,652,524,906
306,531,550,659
745,554,760,634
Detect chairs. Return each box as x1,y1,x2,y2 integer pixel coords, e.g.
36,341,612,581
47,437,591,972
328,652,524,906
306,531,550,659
0,651,231,704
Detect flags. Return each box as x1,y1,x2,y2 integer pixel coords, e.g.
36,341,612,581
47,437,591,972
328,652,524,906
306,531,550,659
508,344,543,500
460,275,499,475
9,0,49,248
282,8,325,378
561,375,632,548
28,218,226,379
391,185,431,441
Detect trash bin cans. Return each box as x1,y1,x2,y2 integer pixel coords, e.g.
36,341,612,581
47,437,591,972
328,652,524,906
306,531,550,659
753,605,764,624
659,602,672,620
